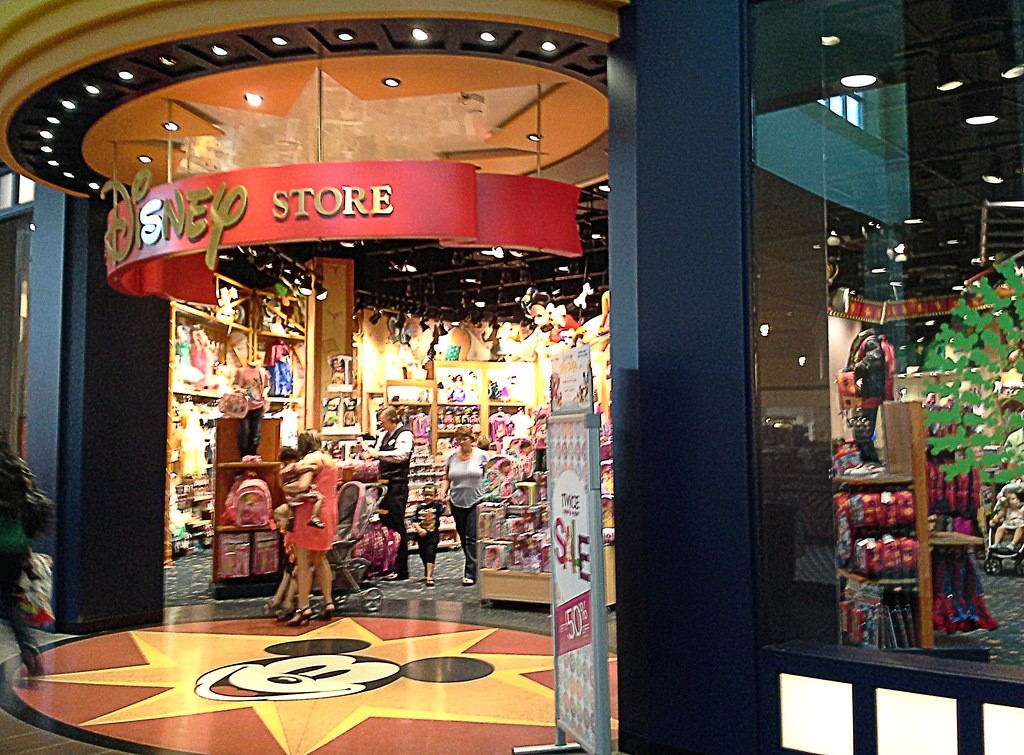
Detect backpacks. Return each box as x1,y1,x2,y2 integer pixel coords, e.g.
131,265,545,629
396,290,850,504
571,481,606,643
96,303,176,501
860,334,895,403
220,478,278,533
481,436,536,503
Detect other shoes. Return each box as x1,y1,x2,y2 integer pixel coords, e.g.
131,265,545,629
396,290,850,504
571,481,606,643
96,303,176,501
17,664,46,690
460,576,474,586
1007,543,1016,551
988,543,1002,549
262,599,296,621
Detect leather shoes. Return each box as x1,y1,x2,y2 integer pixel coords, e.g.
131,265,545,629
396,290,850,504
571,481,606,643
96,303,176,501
382,571,410,581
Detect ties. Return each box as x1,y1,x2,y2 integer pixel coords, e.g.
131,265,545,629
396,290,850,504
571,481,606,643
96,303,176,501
383,432,391,445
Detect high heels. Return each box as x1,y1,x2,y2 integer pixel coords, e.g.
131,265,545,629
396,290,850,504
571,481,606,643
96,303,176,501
315,600,337,620
287,607,315,626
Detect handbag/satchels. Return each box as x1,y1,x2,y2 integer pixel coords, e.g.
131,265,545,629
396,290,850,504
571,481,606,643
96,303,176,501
11,551,56,627
21,489,57,542
217,388,250,418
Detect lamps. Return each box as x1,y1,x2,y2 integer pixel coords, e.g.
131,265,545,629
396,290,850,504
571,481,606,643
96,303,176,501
299,275,327,301
393,252,420,273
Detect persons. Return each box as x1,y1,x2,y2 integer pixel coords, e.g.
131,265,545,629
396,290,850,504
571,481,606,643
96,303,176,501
437,426,491,587
834,326,885,475
411,483,448,587
0,439,44,689
357,407,416,581
233,361,268,463
989,492,1024,551
1000,411,1024,481
263,430,338,628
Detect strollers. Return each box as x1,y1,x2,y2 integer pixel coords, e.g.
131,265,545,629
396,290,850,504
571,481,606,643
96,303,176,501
982,480,1024,575
309,482,388,620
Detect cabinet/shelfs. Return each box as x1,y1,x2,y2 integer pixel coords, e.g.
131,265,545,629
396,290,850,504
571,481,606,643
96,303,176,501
164,273,308,571
475,446,616,608
832,399,1002,664
319,355,364,435
384,333,614,553
207,417,283,601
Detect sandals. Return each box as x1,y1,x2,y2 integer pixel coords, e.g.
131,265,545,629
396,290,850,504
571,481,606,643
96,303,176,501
307,515,325,529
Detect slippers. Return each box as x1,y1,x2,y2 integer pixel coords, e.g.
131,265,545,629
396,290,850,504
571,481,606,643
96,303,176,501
422,575,435,586
